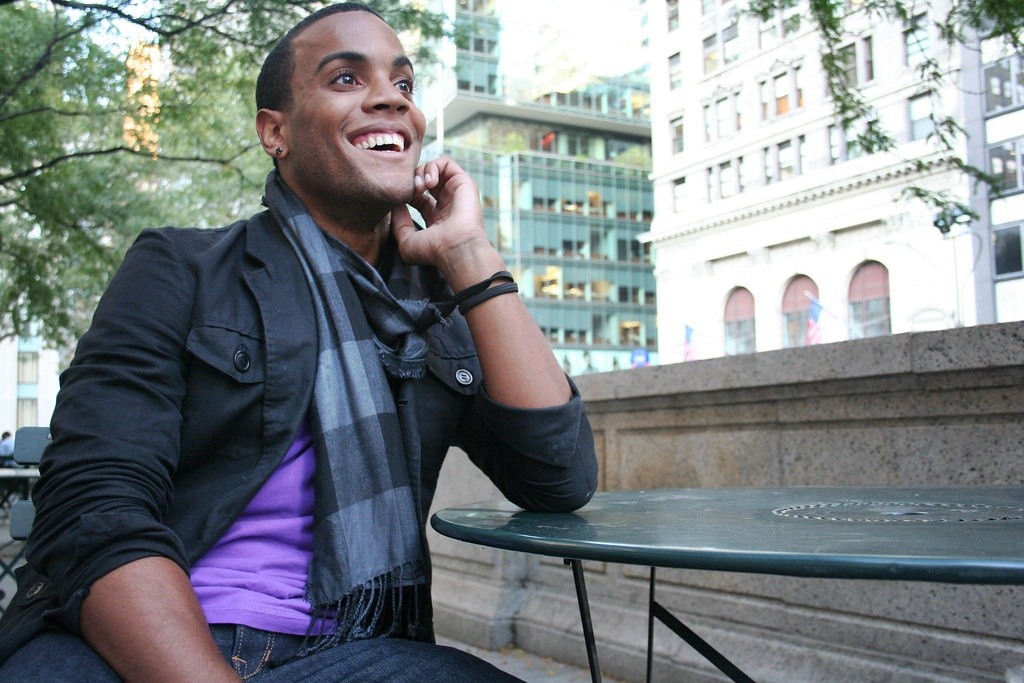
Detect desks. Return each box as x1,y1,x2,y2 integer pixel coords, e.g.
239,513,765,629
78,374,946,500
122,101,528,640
0,468,41,508
430,484,1024,683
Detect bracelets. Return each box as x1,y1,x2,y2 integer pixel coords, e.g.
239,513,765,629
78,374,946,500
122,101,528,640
452,270,518,315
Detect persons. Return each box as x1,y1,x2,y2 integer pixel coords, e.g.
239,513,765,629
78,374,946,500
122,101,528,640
1,431,24,468
0,0,599,682
